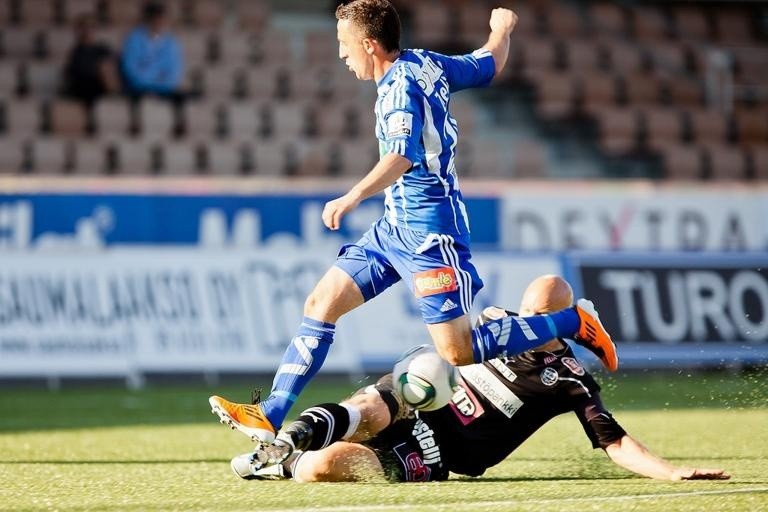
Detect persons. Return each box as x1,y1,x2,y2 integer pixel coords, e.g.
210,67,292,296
231,275,729,483
58,11,122,133
119,0,203,106
209,0,617,444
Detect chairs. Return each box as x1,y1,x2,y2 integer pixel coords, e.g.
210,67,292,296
1,1,768,182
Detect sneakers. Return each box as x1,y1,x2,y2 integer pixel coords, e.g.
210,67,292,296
208,395,283,443
230,431,295,480
570,299,618,373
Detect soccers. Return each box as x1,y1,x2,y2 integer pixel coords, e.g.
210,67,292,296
393,345,458,411
